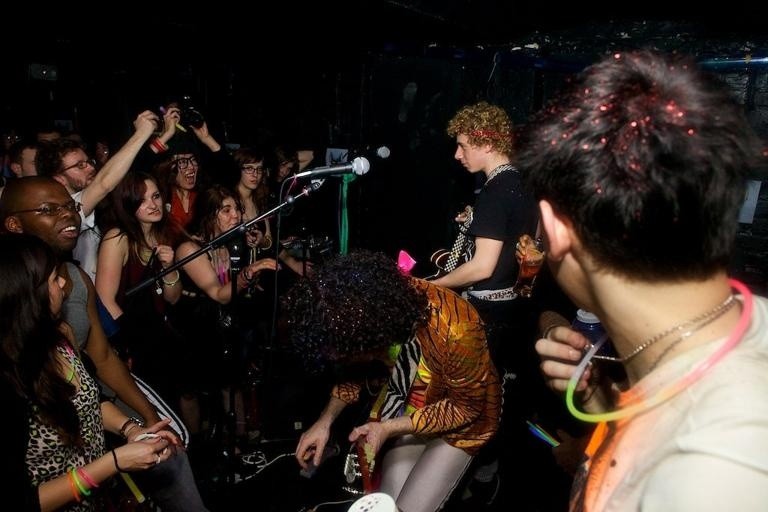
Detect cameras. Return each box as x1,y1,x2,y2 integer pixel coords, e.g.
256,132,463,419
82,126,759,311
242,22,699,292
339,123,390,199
149,108,165,135
177,92,202,130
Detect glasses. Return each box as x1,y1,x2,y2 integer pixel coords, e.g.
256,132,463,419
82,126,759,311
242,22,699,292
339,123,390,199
177,156,202,170
60,159,96,173
243,165,266,174
13,201,82,217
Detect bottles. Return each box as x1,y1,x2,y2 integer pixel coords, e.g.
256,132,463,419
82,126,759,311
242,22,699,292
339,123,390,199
572,309,611,394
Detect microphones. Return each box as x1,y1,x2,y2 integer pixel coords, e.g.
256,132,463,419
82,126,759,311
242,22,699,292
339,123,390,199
348,138,392,165
292,155,371,185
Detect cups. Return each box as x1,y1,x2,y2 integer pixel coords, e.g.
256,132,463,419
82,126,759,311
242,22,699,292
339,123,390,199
513,236,545,299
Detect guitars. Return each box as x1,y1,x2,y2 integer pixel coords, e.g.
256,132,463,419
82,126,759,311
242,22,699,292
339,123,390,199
422,206,473,282
343,383,390,500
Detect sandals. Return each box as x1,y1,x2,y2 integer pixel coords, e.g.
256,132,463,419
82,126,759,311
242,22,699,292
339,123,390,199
230,433,248,455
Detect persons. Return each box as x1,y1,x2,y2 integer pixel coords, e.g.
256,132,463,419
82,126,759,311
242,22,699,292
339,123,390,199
1,47,767,512
